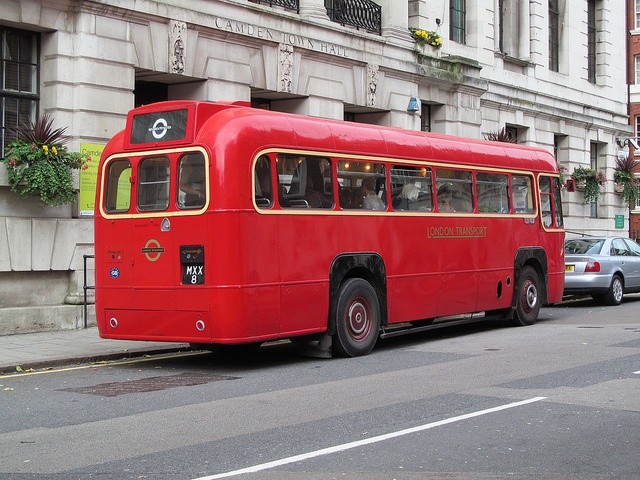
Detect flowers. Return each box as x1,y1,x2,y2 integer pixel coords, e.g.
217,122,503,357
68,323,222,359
0,138,92,210
571,164,608,207
408,27,444,48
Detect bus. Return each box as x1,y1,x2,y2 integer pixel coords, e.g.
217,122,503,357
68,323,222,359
92,98,567,359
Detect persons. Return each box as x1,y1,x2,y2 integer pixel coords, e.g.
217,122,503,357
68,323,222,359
179,153,205,206
362,175,387,210
256,153,475,214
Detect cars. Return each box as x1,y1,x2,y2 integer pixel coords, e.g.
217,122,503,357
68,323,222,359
563,235,639,305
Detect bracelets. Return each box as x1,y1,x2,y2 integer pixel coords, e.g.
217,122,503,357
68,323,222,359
199,192,202,199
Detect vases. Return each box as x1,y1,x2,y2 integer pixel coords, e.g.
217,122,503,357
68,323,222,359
574,175,587,189
613,182,625,193
